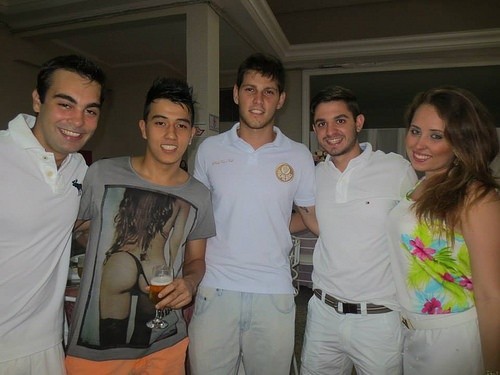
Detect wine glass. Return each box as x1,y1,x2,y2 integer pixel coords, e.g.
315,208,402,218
146,265,174,329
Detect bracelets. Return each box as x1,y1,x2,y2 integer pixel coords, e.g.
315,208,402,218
485,368,500,375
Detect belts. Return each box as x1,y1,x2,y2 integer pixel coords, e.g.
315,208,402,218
401,308,474,331
314,289,393,315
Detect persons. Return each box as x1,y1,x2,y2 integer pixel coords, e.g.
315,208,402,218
385,84,500,375
64,77,217,375
192,51,320,375
0,54,107,375
289,86,418,375
